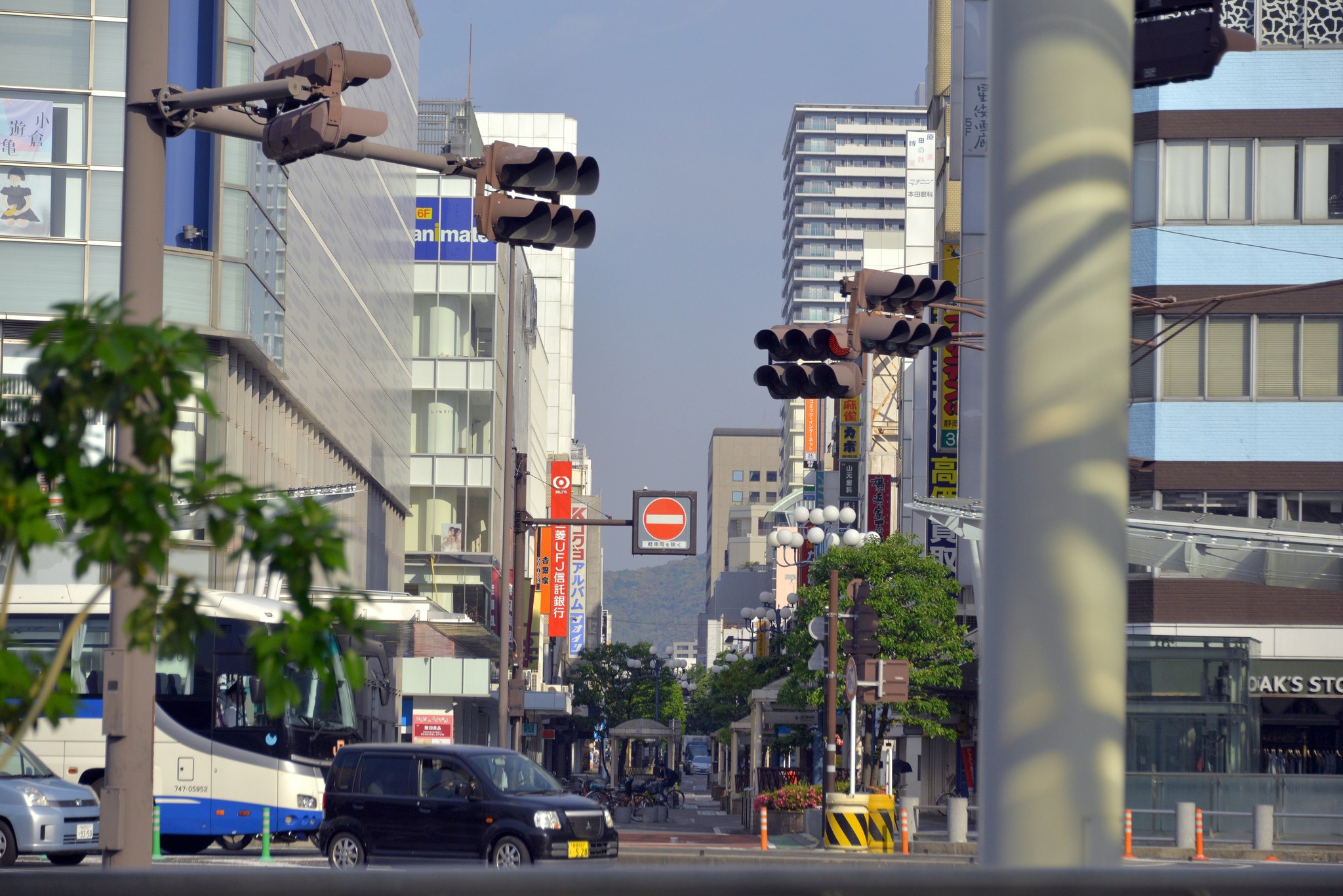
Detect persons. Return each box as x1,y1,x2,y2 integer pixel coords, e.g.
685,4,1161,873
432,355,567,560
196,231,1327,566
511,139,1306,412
606,748,611,762
367,760,396,794
655,763,676,803
222,681,248,727
892,763,902,804
428,766,463,798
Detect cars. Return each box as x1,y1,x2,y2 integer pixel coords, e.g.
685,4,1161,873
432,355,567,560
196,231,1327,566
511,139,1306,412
687,755,713,776
1,726,100,869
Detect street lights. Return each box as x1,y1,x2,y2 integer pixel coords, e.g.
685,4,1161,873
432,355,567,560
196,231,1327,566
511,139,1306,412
740,592,798,654
767,506,862,837
627,646,687,763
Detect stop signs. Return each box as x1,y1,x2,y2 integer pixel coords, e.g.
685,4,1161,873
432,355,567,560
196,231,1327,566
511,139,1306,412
643,497,688,542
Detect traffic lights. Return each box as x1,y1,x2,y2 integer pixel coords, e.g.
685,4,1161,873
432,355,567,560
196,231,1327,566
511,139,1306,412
486,191,597,250
852,310,954,359
753,324,862,362
857,269,958,316
491,140,600,196
753,361,864,399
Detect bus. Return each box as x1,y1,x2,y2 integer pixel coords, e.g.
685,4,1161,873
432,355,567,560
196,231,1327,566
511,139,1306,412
1,583,393,856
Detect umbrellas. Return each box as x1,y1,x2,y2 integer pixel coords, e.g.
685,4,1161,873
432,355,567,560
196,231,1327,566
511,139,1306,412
892,758,913,773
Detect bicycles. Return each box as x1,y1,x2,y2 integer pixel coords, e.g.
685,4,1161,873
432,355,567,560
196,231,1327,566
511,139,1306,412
558,773,685,823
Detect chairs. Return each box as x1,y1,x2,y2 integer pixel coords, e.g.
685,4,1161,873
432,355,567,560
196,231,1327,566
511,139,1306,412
86,669,182,694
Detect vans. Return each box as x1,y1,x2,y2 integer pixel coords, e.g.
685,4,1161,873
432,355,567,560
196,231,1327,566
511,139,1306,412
320,742,621,873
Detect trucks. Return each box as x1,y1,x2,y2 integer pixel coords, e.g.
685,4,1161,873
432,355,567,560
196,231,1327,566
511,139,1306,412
681,735,711,772
